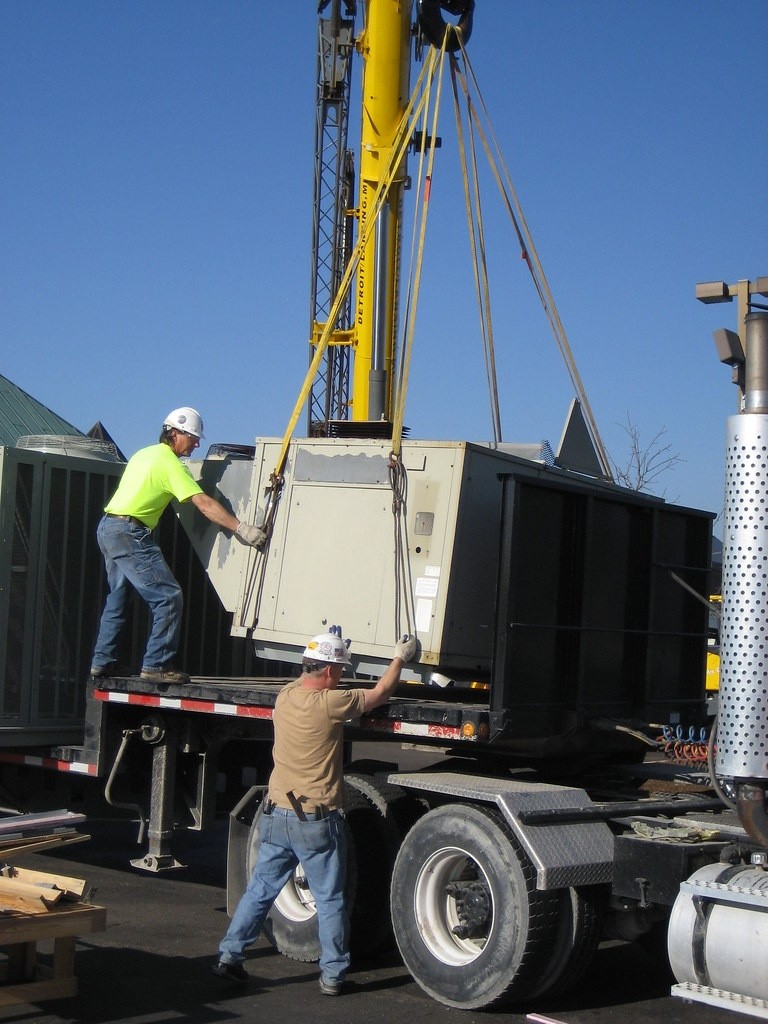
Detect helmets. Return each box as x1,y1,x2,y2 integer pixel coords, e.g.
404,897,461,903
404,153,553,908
303,633,352,665
162,407,206,439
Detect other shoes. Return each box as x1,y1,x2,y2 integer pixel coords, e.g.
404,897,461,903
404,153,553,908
209,960,252,985
140,670,190,683
91,664,133,677
319,975,340,995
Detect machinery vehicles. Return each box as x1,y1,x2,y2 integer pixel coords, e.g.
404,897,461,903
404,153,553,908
1,1,767,1011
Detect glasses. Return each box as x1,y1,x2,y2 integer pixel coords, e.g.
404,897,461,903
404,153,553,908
184,431,199,443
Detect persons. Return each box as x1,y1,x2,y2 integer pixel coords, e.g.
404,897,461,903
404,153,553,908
210,625,416,996
90,406,268,684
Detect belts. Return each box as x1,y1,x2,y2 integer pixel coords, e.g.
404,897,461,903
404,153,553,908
103,513,148,531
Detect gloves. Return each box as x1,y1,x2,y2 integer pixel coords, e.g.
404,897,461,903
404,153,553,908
394,634,416,664
329,625,352,650
234,521,267,551
